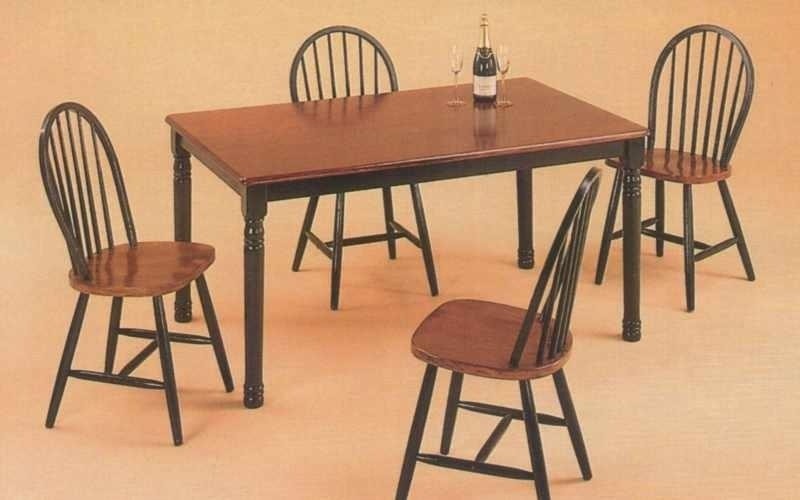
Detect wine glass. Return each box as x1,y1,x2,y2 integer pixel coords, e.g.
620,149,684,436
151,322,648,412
448,46,464,106
496,45,511,105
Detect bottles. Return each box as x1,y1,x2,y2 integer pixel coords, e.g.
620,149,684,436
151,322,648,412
472,12,496,101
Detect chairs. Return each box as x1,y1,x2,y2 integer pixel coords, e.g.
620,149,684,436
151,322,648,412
39,102,234,444
289,25,439,309
397,168,604,499
595,23,755,311
